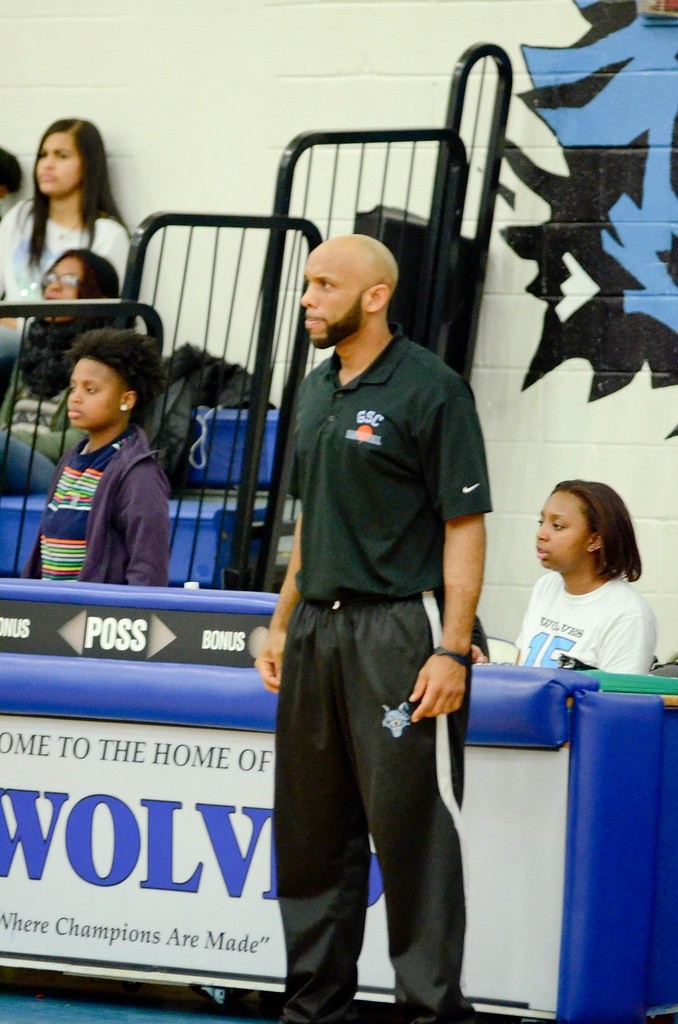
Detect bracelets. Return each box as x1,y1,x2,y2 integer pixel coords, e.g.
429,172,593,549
434,647,470,666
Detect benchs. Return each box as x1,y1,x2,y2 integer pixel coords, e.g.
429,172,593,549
0,407,298,586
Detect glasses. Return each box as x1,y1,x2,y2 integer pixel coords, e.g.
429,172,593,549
42,273,84,288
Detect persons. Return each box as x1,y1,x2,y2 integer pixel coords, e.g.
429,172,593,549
21,327,169,587
471,613,489,664
0,249,118,494
515,480,659,673
255,234,492,1024
0,120,129,393
